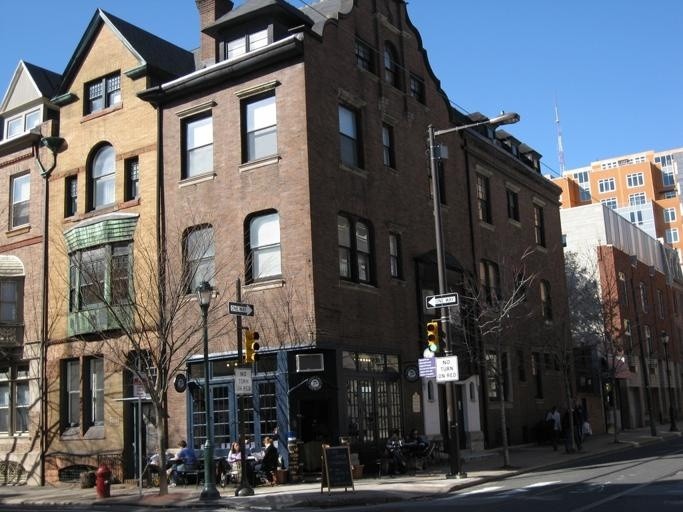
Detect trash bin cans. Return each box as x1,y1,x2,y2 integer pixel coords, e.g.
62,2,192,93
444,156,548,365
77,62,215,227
79,471,95,489
607,409,621,434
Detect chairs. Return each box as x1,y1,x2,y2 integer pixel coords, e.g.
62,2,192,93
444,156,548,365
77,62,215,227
146,455,280,486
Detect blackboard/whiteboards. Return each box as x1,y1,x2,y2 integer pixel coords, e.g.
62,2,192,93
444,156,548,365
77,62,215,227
324,446,353,488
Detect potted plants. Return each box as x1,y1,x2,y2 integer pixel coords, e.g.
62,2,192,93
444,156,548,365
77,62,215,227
276,456,289,483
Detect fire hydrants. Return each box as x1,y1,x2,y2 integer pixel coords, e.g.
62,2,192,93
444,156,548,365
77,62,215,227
94,463,112,499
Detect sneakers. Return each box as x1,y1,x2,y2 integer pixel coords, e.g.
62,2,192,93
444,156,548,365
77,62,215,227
168,483,176,487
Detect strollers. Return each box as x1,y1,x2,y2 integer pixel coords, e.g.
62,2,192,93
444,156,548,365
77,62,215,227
147,450,186,488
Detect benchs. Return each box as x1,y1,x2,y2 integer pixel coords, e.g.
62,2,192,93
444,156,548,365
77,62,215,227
373,442,435,478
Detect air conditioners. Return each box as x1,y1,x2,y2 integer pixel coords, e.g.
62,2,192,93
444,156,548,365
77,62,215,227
295,353,326,373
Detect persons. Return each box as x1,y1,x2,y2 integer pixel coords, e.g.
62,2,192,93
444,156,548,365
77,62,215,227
386,428,426,474
135,440,196,488
228,436,278,486
545,395,588,455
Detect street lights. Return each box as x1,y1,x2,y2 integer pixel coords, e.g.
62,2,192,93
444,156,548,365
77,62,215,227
29,134,68,485
424,109,522,478
660,328,680,433
193,278,223,501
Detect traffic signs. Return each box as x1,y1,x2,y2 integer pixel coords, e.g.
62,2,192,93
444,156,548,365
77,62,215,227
426,292,460,309
228,300,255,317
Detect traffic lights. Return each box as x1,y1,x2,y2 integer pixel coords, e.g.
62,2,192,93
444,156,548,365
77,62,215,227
426,321,441,353
245,328,261,362
